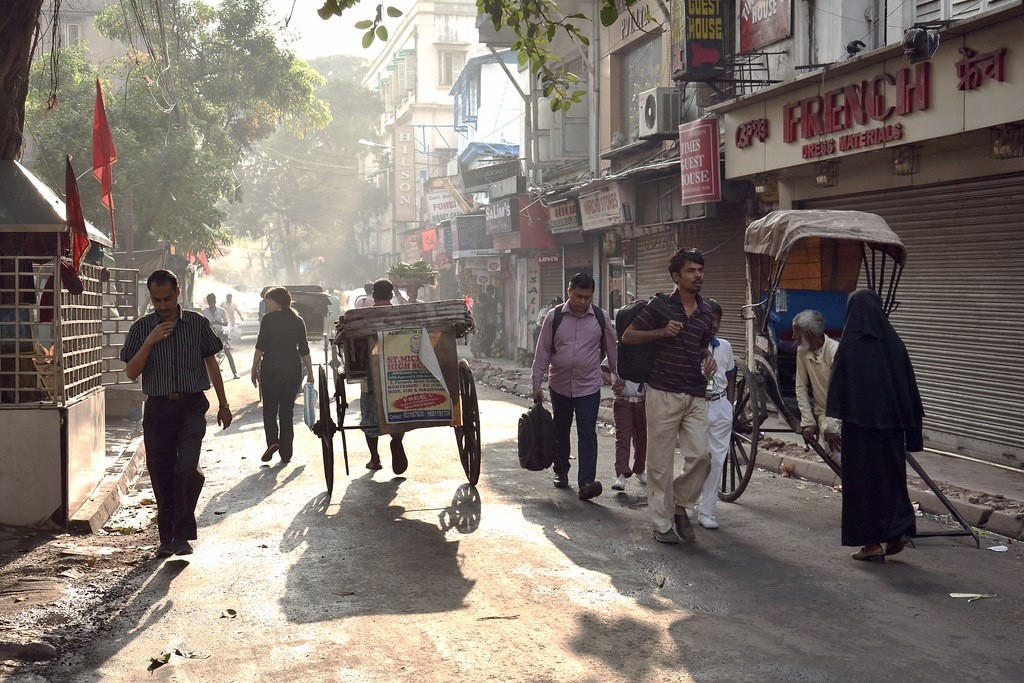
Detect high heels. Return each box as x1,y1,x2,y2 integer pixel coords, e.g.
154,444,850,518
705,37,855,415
851,547,885,563
885,536,908,555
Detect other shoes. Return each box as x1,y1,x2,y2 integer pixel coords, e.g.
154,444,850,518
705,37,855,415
281,451,294,462
174,538,194,556
390,439,408,474
159,540,173,556
260,440,280,462
367,454,382,470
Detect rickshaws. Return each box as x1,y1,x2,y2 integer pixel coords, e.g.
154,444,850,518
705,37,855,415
713,207,984,544
313,298,482,485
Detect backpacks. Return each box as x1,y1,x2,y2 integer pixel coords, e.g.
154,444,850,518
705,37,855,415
616,291,680,383
518,397,558,472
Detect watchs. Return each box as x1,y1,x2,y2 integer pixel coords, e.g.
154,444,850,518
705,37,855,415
219,403,230,409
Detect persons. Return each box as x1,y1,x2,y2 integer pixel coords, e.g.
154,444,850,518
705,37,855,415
470,292,488,357
481,284,499,356
791,309,845,471
598,353,647,492
527,272,624,500
351,277,412,474
118,269,233,556
619,248,717,543
694,297,737,529
250,287,316,463
176,258,433,378
531,294,564,355
823,289,926,563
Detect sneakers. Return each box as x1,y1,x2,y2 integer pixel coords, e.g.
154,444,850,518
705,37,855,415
553,472,568,487
636,472,647,483
698,512,718,528
674,513,696,542
578,481,602,500
654,529,678,543
610,474,625,491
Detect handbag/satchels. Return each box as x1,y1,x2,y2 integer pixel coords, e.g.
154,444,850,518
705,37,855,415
303,381,317,429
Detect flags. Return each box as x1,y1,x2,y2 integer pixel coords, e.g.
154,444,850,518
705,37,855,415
63,152,91,275
88,79,118,208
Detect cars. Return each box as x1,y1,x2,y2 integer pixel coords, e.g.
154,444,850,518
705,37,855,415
337,287,425,314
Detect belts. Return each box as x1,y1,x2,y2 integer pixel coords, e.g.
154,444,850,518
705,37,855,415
148,392,203,401
705,390,725,402
614,395,645,403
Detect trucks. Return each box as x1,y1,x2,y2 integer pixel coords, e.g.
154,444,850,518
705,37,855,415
258,285,333,342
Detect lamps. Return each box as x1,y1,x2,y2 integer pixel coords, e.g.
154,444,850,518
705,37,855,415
901,16,969,64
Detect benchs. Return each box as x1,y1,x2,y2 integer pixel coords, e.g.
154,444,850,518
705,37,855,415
759,289,867,355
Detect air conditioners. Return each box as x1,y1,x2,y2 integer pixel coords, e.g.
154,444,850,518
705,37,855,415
638,86,682,138
